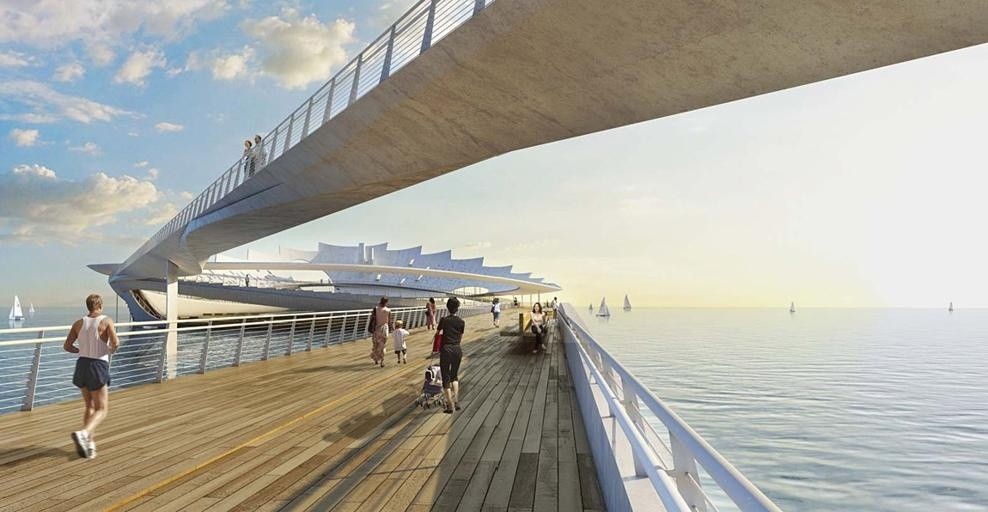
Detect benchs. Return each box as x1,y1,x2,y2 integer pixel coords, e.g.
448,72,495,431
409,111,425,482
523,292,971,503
500,311,557,351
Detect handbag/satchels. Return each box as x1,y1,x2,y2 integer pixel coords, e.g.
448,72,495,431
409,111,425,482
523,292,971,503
431,316,446,353
368,307,377,334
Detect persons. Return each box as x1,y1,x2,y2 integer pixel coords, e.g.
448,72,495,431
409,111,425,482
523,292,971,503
551,297,558,318
370,297,392,367
242,140,255,176
63,295,119,459
530,302,548,354
513,297,518,306
255,135,267,167
392,320,409,363
425,297,437,330
490,298,500,327
431,296,464,413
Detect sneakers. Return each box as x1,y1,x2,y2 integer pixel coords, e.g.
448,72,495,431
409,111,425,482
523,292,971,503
87,440,96,459
72,429,90,457
442,402,462,414
